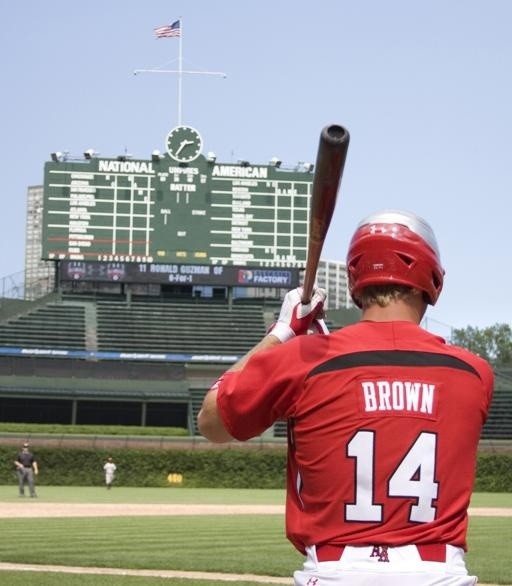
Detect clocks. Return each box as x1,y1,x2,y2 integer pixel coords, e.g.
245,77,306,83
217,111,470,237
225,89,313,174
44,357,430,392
166,126,202,163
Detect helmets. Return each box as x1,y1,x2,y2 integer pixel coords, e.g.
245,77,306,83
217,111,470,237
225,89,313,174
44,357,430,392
346,209,444,309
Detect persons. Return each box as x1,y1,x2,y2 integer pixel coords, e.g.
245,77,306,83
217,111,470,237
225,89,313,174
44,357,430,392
103,456,118,490
195,208,497,586
12,443,40,497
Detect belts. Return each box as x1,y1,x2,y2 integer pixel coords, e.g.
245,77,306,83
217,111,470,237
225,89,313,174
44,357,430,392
314,539,447,564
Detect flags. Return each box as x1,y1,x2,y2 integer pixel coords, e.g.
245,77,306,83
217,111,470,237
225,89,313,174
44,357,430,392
154,20,180,38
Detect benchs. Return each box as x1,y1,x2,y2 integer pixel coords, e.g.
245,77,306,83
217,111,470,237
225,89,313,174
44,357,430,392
0,301,343,436
481,391,511,439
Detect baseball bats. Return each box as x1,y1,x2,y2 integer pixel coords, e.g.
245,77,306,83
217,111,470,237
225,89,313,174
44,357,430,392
301,125,349,305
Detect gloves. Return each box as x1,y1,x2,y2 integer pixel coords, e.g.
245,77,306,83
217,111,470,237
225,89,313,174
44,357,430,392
266,286,329,343
